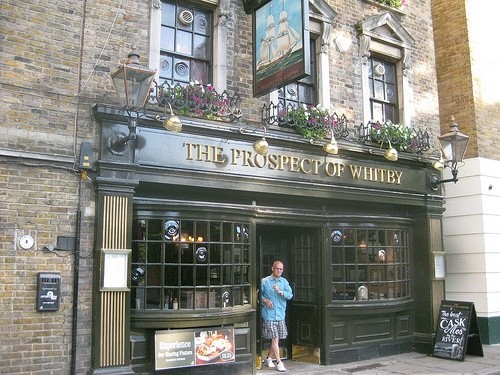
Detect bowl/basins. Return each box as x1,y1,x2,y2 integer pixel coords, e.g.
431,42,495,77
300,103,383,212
196,342,232,362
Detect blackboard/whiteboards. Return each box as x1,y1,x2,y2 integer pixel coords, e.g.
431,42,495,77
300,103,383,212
431,299,484,363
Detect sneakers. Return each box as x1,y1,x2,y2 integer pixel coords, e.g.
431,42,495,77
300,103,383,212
276,361,286,371
265,358,275,367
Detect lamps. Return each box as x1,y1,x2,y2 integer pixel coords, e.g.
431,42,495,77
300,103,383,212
310,128,339,154
251,124,270,155
428,112,471,191
428,149,445,171
163,101,183,134
109,39,158,147
380,138,398,161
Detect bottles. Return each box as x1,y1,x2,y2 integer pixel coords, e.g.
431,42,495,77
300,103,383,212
173,298,178,309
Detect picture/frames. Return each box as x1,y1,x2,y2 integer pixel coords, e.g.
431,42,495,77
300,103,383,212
348,268,366,282
371,268,384,286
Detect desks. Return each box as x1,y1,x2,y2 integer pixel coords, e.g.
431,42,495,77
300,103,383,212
179,290,218,308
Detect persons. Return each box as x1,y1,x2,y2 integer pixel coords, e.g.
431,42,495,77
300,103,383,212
261,261,294,372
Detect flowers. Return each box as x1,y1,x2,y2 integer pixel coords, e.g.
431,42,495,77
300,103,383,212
369,118,412,150
376,0,413,14
165,80,242,122
280,103,338,139
